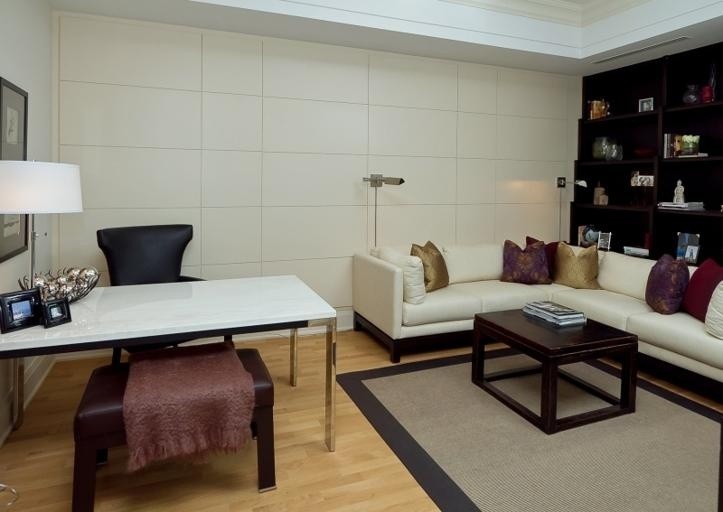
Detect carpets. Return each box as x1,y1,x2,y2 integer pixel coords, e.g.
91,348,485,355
332,341,721,512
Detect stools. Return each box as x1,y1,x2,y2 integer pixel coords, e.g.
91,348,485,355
62,346,278,512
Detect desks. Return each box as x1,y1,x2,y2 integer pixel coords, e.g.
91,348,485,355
0,270,344,457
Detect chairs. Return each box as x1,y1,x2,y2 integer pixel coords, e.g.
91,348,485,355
93,224,236,365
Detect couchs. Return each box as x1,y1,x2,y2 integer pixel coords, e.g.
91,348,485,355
349,237,723,413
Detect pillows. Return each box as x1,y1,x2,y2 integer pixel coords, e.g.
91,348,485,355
553,241,604,291
376,246,427,308
678,257,723,323
524,236,567,282
497,239,553,286
703,276,723,341
408,240,449,292
642,252,691,316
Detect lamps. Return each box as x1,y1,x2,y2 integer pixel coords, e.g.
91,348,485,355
0,157,83,291
554,173,588,240
363,172,406,250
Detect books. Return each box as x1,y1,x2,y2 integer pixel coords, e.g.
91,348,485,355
521,299,586,326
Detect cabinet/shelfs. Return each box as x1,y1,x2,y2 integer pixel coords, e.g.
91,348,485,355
568,41,722,274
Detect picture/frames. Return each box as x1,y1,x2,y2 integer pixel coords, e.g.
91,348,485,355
0,286,45,334
38,294,71,329
0,75,28,264
